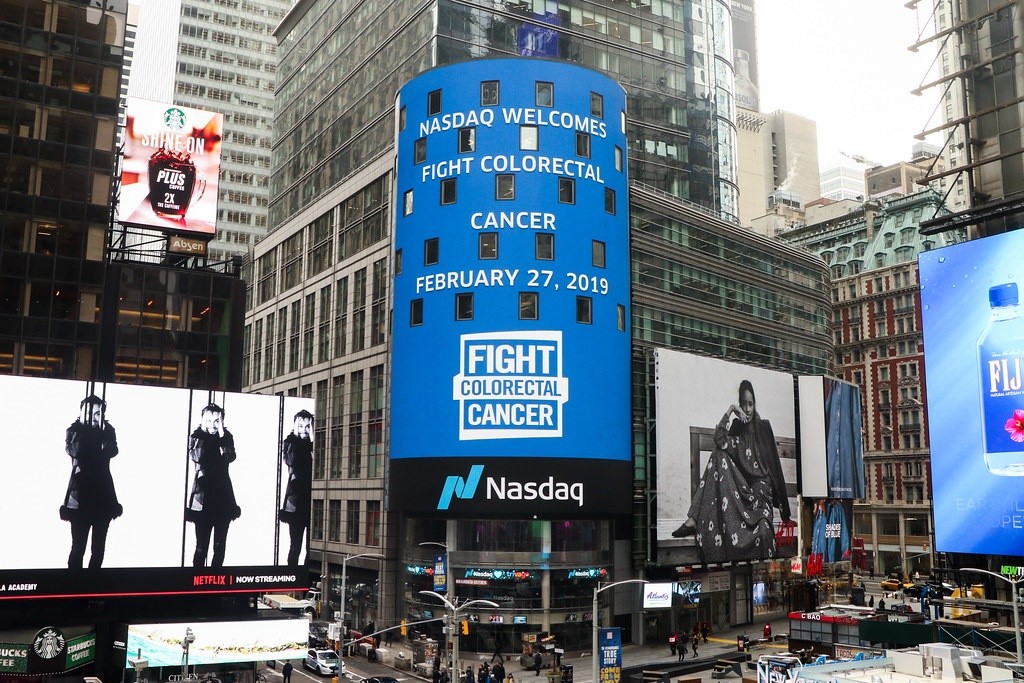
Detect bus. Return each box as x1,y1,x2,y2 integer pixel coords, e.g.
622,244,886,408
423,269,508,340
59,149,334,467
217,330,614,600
263,594,305,619
300,591,322,616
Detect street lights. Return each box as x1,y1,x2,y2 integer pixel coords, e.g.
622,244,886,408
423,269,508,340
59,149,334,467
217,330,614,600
592,580,650,682
960,567,1023,664
338,553,386,681
420,589,500,683
419,542,450,677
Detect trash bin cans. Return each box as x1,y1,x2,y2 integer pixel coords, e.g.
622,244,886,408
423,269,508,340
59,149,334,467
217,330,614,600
368,649,374,662
737,635,750,651
559,664,573,683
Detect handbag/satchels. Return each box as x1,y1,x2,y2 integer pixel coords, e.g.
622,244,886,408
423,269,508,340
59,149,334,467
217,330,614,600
695,645,699,649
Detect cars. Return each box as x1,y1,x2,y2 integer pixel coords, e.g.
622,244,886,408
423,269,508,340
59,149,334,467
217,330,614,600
928,582,958,596
904,584,938,597
360,677,398,683
810,574,864,588
302,647,346,675
882,579,915,590
309,622,334,648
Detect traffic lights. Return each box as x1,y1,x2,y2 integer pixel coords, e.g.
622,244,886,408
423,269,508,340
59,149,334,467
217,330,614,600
461,620,468,635
923,544,926,551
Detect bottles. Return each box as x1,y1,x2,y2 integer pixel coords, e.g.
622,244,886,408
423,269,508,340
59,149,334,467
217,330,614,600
977,283,1024,476
148,107,196,220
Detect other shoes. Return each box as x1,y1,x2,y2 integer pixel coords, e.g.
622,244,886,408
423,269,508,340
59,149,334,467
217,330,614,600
672,525,696,536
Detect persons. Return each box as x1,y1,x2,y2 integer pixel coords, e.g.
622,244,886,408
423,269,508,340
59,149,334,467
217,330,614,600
282,660,293,683
701,624,708,643
869,596,874,606
908,570,920,582
433,661,515,683
671,632,698,661
868,566,874,580
364,621,392,648
60,394,122,569
811,497,850,562
187,404,241,568
879,598,885,609
489,642,504,663
671,380,797,562
535,653,542,675
279,409,314,565
